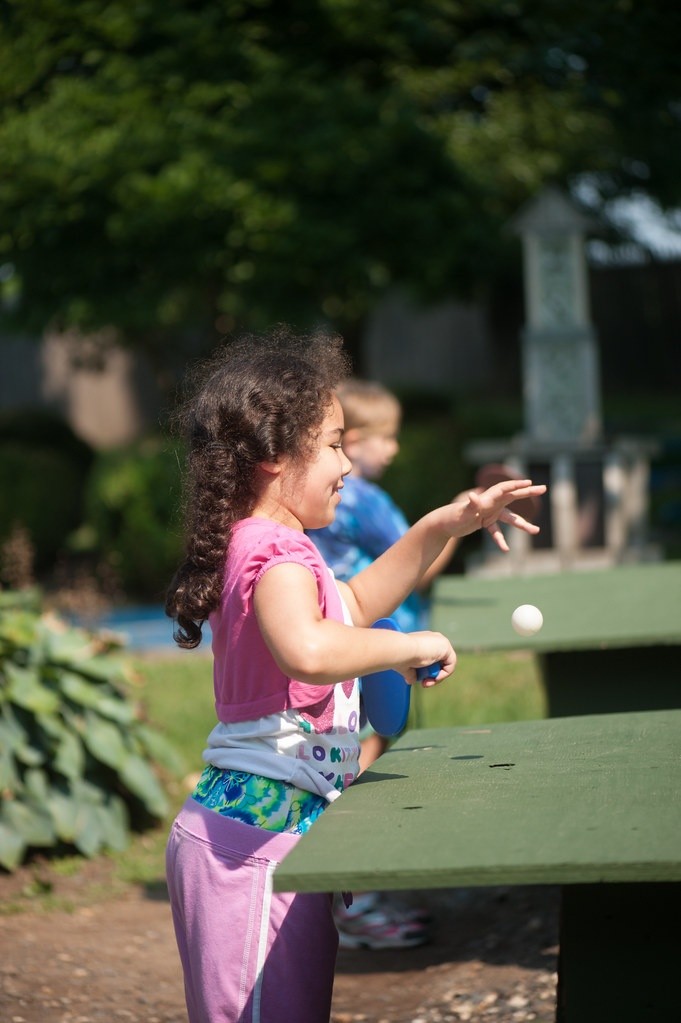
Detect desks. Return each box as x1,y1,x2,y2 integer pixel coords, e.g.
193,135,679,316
272,708,681,1023
433,560,680,718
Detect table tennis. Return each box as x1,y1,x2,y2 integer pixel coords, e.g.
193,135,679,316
510,604,543,637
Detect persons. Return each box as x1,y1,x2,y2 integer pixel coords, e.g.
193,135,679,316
165,325,547,1023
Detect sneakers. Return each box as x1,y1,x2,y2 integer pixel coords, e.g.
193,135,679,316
333,897,421,950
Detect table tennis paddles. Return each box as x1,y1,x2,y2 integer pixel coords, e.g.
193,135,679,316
361,617,443,739
477,467,542,523
